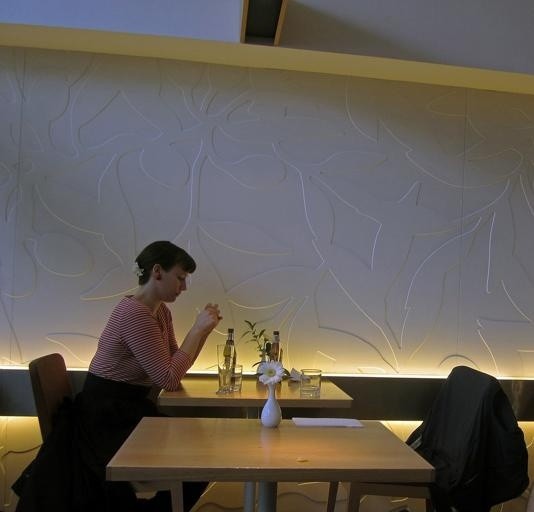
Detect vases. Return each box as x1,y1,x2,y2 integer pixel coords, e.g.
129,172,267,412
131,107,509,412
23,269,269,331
261,383,282,428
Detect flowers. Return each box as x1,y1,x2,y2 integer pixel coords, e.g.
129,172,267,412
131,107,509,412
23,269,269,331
258,361,285,385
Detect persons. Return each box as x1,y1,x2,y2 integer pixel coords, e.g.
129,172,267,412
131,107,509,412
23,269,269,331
68,237,221,510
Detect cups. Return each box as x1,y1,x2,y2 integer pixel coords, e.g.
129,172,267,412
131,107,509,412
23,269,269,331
230,365,243,392
216,344,233,395
301,369,321,396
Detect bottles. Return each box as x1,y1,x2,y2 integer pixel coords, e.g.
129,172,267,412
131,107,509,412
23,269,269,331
224,328,236,386
270,331,283,363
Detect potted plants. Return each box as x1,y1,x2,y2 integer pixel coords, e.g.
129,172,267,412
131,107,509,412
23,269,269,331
241,319,272,383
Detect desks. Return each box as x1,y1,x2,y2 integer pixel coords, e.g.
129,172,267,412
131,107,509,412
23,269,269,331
158,378,354,512
106,417,436,512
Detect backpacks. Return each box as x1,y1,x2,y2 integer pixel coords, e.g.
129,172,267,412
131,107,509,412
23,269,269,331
406,366,530,512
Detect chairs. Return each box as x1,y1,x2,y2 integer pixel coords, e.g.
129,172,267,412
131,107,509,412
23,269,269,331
342,365,499,512
28,353,183,512
